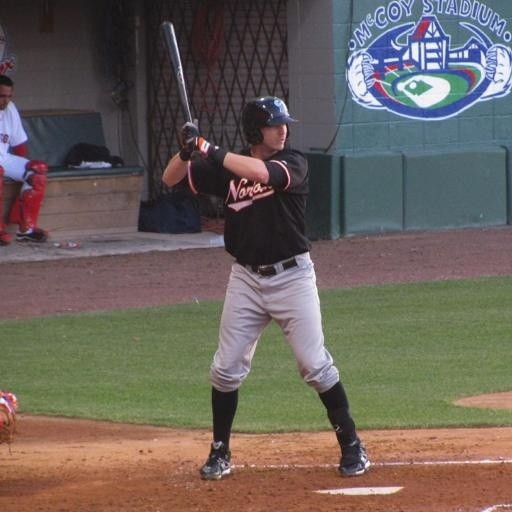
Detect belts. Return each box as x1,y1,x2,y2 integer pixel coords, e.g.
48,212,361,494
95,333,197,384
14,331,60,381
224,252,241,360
234,258,298,279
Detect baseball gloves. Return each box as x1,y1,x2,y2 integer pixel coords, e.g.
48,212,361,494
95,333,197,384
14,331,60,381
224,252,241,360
0,392,17,445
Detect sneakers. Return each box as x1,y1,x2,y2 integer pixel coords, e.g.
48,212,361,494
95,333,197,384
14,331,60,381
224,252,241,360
199,441,233,480
339,439,371,477
16,227,47,243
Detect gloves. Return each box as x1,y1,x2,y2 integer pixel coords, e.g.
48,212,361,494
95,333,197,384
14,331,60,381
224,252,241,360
178,119,208,160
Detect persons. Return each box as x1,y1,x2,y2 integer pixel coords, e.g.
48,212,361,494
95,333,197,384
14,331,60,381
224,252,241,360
161,94,371,481
0,75,50,245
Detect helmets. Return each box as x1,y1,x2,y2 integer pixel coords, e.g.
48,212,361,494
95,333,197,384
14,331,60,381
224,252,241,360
241,95,299,145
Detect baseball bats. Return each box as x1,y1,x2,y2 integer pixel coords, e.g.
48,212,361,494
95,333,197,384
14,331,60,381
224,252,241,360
160,20,204,163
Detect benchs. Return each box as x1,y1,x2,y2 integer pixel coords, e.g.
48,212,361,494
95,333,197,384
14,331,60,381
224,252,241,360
0,109,146,242
